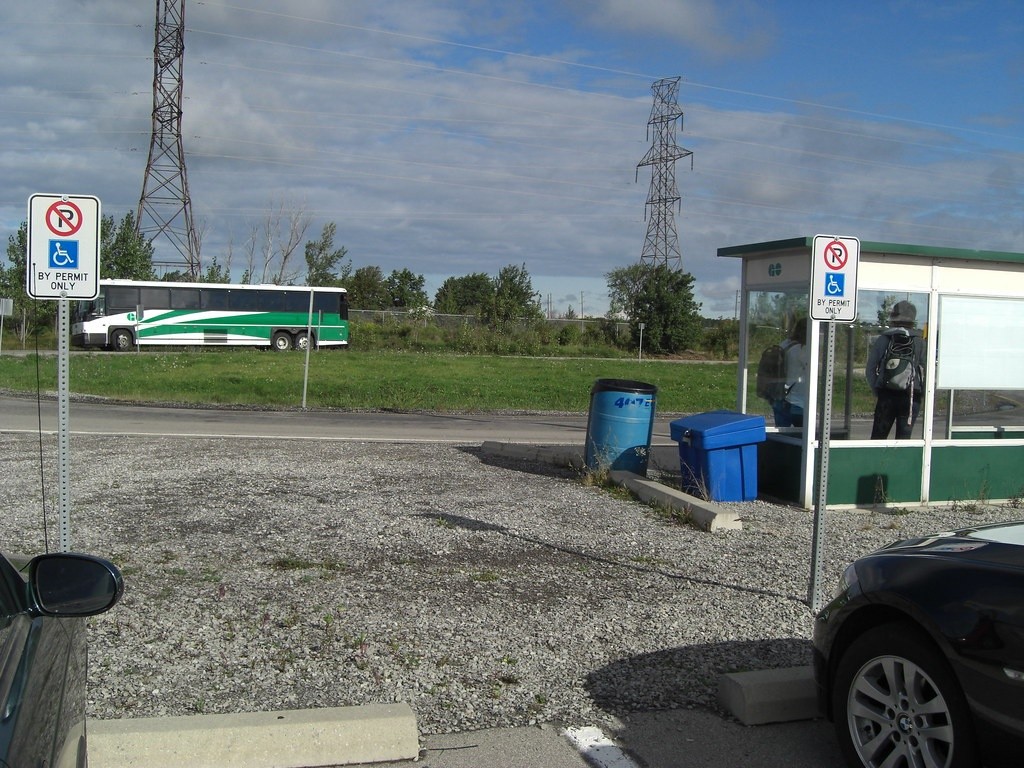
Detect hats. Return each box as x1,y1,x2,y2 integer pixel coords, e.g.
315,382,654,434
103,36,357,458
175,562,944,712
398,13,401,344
886,300,917,323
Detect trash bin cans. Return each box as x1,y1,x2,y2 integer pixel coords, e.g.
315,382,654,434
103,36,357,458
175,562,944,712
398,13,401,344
584,379,658,477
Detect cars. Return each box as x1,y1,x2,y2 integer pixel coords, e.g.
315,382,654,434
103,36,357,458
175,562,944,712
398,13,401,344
0,546,126,768
811,519,1024,768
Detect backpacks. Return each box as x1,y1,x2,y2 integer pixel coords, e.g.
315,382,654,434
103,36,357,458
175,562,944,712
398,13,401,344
757,342,800,406
875,333,917,391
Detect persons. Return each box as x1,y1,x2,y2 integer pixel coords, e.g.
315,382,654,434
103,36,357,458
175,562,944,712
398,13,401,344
772,318,807,427
866,301,927,440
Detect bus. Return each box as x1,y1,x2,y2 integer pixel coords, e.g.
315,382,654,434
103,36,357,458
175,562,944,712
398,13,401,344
70,277,351,354
